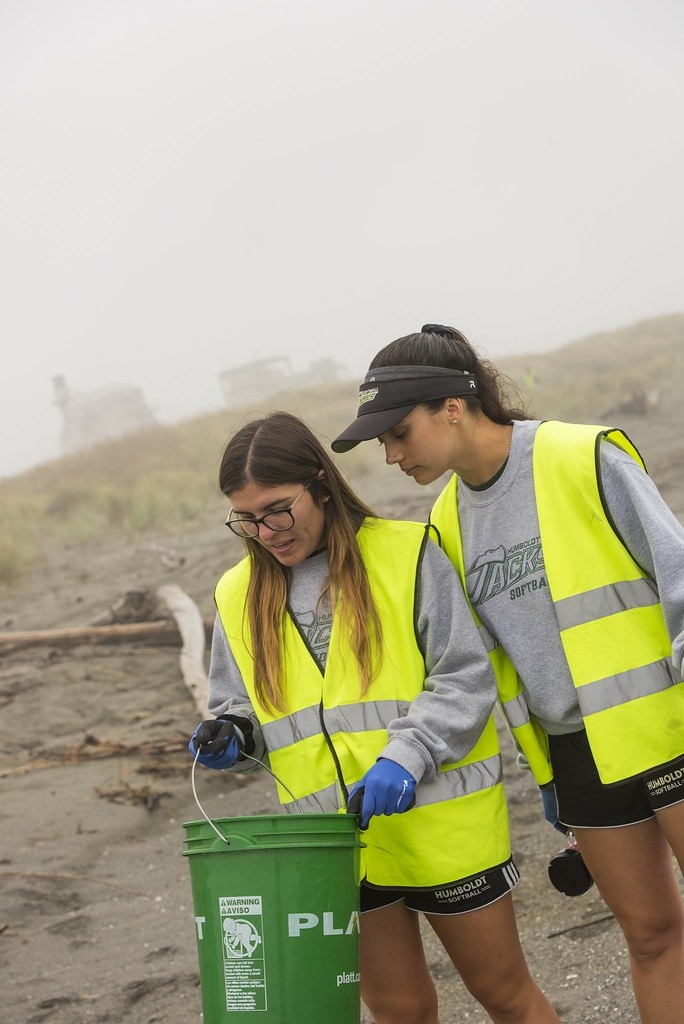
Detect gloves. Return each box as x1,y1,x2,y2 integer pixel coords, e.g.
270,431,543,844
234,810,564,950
346,759,416,831
188,714,256,769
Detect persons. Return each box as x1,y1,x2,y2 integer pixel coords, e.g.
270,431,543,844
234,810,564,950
187,412,561,1024
332,325,684,1024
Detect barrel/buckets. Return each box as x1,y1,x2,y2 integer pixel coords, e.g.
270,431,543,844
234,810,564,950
179,741,366,1024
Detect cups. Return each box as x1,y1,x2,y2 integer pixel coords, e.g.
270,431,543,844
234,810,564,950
547,830,594,897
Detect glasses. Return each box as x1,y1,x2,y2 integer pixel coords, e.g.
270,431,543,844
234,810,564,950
224,480,315,539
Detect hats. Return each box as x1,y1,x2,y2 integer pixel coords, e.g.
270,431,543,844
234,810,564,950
331,364,479,453
